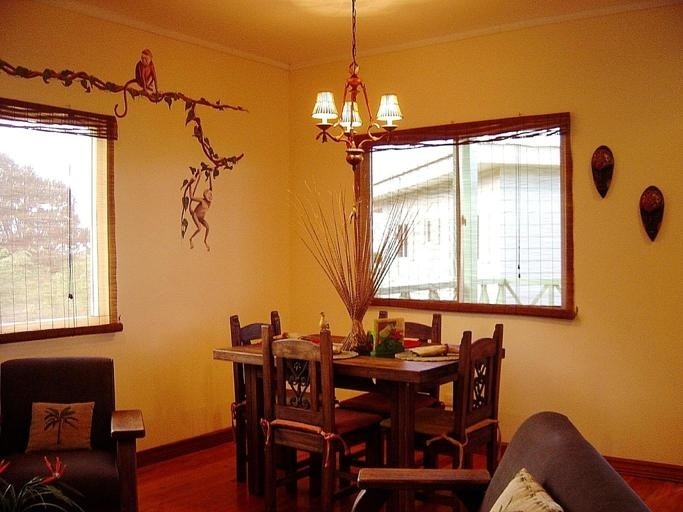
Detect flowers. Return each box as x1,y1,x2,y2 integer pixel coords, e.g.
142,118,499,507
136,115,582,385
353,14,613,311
0,454,84,512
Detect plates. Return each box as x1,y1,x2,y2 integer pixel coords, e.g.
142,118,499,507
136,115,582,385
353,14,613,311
332,350,359,360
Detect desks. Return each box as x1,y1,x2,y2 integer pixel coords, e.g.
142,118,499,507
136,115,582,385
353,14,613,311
212,341,505,511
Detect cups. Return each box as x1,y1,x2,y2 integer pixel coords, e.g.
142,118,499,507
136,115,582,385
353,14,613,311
333,343,343,353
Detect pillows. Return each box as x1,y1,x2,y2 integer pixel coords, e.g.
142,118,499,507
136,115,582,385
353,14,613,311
24,401,96,454
489,467,563,511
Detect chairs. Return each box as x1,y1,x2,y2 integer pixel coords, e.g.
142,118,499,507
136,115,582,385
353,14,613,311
351,410,652,511
341,311,441,494
227,310,282,482
380,324,503,511
260,325,380,511
0,356,145,512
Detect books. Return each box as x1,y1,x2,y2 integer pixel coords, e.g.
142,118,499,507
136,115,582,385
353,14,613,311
371,316,406,353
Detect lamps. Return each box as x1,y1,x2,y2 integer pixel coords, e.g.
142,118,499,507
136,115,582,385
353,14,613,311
309,1,404,172
